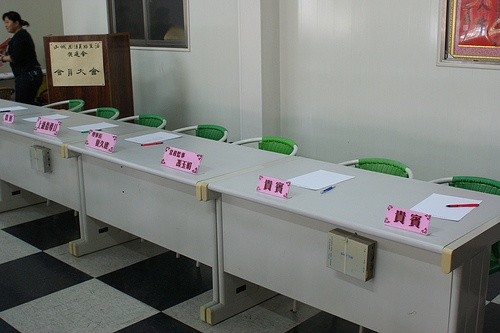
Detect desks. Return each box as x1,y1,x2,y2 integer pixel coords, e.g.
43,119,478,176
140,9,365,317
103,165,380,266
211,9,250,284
0,69,47,101
65,129,290,321
0,98,152,255
201,156,500,333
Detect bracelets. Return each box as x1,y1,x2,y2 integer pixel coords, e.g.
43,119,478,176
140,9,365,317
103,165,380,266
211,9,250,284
0,55,7,63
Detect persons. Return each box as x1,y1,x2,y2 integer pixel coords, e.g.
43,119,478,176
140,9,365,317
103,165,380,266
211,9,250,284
0,11,43,106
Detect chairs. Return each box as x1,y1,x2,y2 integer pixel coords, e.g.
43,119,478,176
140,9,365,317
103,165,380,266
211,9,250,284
44,100,500,333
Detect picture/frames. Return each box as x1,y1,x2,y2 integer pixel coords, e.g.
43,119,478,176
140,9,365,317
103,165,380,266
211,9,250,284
437,0,500,70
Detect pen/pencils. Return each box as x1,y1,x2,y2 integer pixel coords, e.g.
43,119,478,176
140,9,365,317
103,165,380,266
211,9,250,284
142,141,163,146
447,204,479,208
0,110,10,113
81,129,102,133
321,185,336,194
